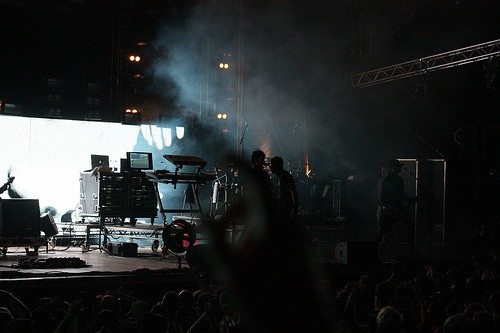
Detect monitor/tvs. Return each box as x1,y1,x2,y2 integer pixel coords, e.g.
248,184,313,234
120,158,128,172
127,152,153,170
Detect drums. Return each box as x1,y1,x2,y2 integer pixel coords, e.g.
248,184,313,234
210,187,237,204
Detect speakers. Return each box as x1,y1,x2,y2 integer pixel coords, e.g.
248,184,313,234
334,241,380,265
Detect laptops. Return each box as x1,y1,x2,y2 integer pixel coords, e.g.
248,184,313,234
84,154,109,172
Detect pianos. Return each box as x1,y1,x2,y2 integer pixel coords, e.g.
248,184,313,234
153,172,216,180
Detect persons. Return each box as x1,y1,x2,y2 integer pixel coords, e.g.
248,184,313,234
250,150,274,199
372,160,419,257
270,156,299,232
212,149,278,250
0,252,500,333
